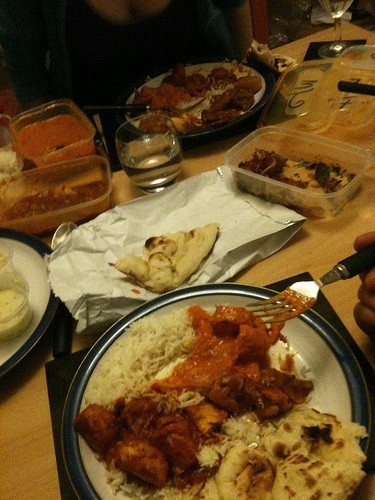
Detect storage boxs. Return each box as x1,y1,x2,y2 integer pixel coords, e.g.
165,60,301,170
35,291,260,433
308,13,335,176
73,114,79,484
0,98,112,232
225,125,369,218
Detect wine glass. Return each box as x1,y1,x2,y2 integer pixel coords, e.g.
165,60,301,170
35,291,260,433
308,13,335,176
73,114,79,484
317,0,352,59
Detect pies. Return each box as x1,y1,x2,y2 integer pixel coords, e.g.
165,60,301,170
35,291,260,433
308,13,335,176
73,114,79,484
216,407,368,500
113,222,219,294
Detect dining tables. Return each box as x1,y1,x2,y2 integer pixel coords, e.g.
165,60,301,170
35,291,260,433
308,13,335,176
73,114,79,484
0,23,375,500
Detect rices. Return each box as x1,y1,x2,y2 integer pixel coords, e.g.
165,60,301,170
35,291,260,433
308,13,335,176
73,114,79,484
84,308,311,500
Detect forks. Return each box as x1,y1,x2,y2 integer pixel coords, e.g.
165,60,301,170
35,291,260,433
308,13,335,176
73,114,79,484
82,97,206,112
247,243,374,329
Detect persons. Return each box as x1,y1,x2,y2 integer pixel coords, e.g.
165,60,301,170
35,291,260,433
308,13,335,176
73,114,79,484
0,1,236,111
354,231,375,339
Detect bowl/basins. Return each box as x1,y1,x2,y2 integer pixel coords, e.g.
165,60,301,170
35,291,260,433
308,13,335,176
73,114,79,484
0,113,23,176
0,156,113,235
222,127,370,219
10,99,97,167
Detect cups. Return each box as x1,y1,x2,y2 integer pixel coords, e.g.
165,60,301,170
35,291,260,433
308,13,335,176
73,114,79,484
115,112,182,195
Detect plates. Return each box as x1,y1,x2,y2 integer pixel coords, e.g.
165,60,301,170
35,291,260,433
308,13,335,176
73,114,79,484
1,228,57,380
118,54,275,140
61,283,375,500
